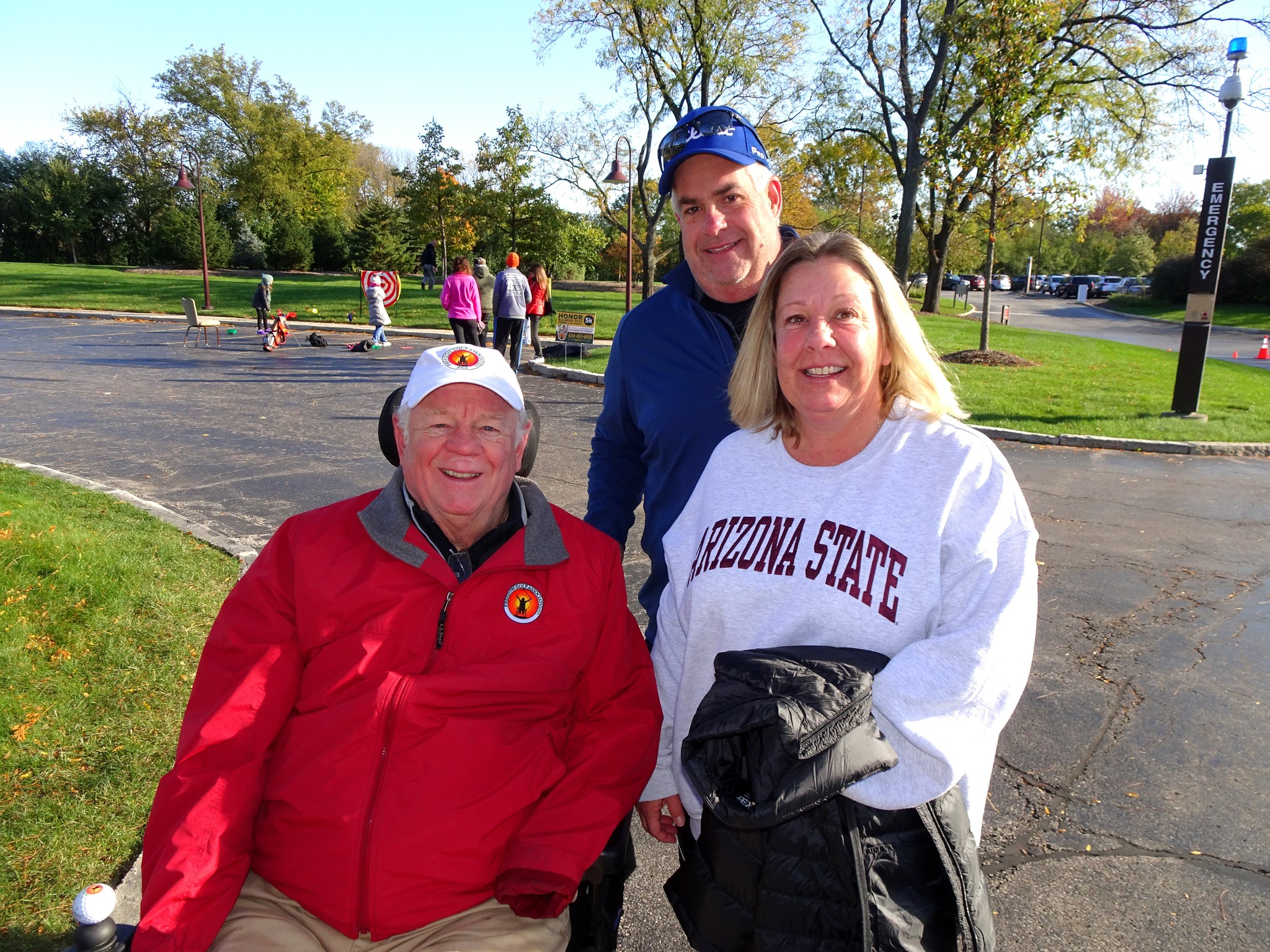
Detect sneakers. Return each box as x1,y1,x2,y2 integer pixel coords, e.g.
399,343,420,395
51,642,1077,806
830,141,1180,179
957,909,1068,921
257,329,268,336
265,329,271,333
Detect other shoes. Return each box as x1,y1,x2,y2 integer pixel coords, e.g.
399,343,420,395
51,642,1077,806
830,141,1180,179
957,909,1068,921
374,342,383,346
383,341,391,346
528,355,545,363
421,283,425,290
428,286,433,290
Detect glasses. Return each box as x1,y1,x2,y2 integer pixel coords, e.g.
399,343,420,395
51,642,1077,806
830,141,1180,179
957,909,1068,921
658,109,769,173
447,548,471,583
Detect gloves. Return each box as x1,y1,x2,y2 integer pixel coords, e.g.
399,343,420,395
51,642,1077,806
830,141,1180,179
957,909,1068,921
526,313,530,320
477,321,485,335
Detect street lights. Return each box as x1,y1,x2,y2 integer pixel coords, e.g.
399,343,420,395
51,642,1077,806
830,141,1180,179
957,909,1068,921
601,136,633,313
171,150,215,310
1033,186,1053,293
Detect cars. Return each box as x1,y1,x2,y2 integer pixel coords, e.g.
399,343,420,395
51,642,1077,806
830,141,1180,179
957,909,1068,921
908,272,1153,297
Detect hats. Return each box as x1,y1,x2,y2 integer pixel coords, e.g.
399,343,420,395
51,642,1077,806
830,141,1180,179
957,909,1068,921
262,273,274,285
399,344,526,418
473,257,486,265
370,272,381,284
506,253,519,268
658,106,771,194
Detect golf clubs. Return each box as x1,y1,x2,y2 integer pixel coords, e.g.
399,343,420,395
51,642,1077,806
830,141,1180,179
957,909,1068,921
270,309,297,321
348,301,369,322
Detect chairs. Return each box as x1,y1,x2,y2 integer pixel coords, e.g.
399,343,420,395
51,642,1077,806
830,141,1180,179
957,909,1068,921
181,296,220,347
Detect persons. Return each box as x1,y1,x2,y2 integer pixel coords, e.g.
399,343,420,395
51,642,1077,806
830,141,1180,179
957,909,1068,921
420,240,438,290
130,344,663,952
252,274,274,336
633,233,1039,952
366,273,392,347
582,105,799,653
441,252,552,375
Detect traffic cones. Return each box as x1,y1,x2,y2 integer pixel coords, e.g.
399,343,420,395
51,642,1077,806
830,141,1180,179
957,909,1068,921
1232,351,1237,358
1254,335,1270,359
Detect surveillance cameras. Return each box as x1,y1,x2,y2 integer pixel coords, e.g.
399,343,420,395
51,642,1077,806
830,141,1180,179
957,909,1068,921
1218,76,1242,111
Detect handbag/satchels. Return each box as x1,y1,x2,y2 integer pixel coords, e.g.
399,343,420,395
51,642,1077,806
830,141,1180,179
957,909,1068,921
524,316,532,345
347,340,373,352
305,333,327,347
544,297,552,316
